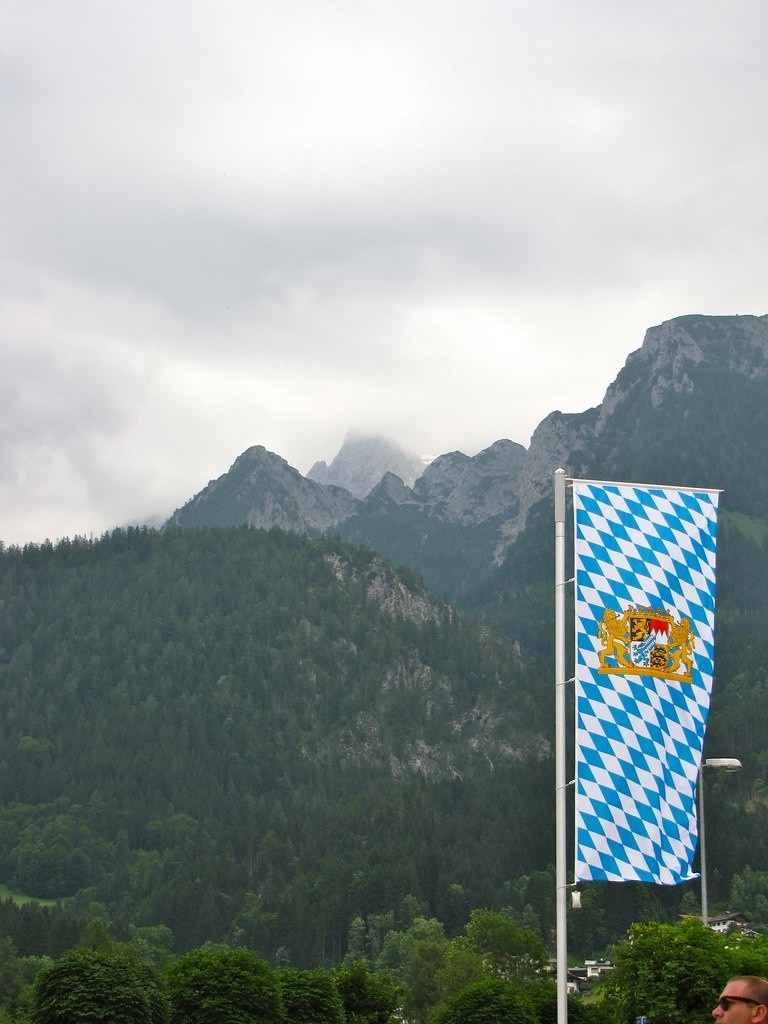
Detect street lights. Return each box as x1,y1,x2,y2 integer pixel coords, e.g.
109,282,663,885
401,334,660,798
697,757,743,929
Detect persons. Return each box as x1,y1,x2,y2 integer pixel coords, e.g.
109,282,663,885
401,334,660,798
712,976,768,1024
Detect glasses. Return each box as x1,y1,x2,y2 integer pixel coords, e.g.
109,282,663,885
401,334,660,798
716,996,761,1011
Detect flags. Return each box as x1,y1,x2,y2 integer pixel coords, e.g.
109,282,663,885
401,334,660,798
572,477,721,887
